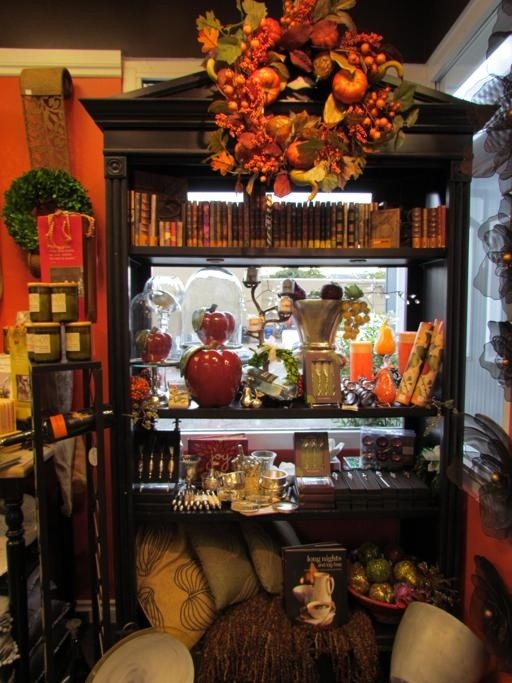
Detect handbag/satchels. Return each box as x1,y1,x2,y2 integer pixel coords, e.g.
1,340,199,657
38,215,86,321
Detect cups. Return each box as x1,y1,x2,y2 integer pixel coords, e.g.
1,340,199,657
292,584,313,602
251,449,277,467
306,601,332,619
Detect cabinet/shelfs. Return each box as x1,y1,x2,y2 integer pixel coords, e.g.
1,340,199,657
100,146,474,650
0,360,107,682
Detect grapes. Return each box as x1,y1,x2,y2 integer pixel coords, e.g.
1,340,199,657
340,286,370,340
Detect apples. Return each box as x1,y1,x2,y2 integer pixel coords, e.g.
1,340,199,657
322,281,345,301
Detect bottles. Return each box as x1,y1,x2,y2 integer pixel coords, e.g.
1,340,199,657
239,385,253,408
203,469,219,493
168,383,191,409
24,279,93,364
348,340,373,383
342,377,356,390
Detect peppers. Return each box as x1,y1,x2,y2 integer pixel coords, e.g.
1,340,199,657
191,303,235,346
331,66,369,101
287,140,314,165
247,18,339,50
265,115,293,142
134,326,172,364
250,68,281,103
178,340,242,408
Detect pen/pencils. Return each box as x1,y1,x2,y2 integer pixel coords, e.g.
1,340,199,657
172,490,222,511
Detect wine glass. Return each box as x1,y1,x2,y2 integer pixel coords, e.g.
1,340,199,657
180,453,201,499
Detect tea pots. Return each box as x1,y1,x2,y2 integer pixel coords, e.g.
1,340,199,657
312,572,334,604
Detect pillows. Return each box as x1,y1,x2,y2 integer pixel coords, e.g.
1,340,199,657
132,520,217,653
186,520,262,610
236,520,303,598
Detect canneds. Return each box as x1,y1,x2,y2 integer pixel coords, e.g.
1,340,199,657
24,323,34,361
64,321,92,359
49,282,78,321
27,282,51,322
34,322,61,362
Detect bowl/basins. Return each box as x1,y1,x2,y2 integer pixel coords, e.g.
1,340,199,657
217,456,297,514
346,580,407,627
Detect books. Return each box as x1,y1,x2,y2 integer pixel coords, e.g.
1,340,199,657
281,541,348,627
129,189,446,250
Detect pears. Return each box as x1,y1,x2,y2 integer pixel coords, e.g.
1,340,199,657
374,324,396,355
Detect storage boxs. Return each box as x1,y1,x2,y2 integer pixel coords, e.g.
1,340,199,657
36,213,98,324
